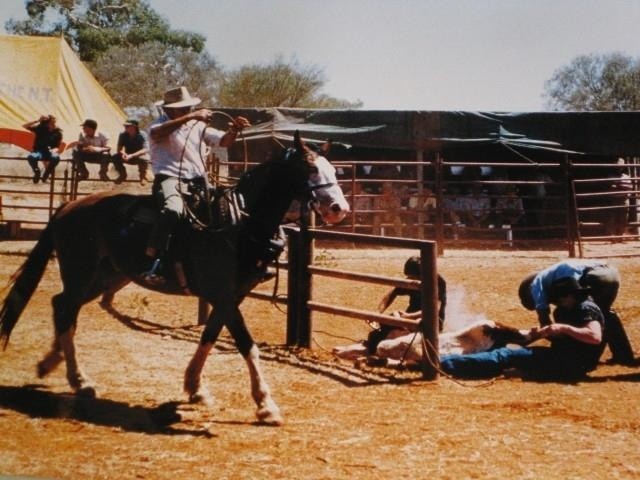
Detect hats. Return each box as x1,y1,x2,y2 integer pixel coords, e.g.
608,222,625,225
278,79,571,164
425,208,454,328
154,86,202,109
122,118,141,128
79,119,97,129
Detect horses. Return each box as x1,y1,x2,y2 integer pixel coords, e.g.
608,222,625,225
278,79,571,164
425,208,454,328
333,311,548,362
0,127,349,426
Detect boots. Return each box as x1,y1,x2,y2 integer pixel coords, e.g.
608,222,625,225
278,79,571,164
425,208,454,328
42,167,54,184
98,166,110,182
31,167,41,184
139,167,150,186
142,207,185,290
77,166,90,181
115,166,128,186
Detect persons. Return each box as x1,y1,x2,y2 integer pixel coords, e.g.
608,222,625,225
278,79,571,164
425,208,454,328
22,114,63,183
72,119,111,182
604,157,633,244
519,258,638,367
333,162,527,230
111,120,148,187
367,256,446,355
136,86,251,287
437,274,605,386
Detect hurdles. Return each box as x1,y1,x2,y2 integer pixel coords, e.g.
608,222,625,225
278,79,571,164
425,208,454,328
199,226,439,379
